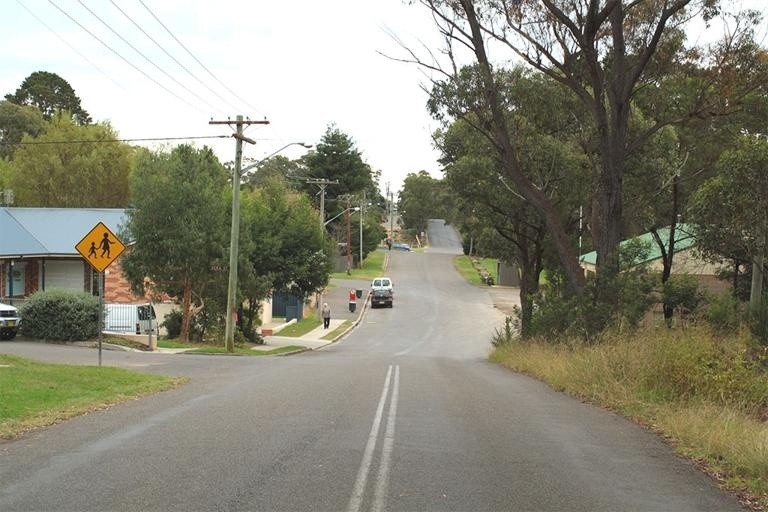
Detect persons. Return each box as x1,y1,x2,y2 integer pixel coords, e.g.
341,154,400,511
348,288,356,312
388,242,392,250
322,302,331,328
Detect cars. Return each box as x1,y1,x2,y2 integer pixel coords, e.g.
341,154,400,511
392,242,410,251
0,302,22,340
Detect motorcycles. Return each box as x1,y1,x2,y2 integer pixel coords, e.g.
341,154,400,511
486,273,492,286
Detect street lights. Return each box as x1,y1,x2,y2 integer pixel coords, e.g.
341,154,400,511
225,143,312,352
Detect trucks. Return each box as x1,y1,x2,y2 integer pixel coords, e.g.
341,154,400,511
370,277,394,308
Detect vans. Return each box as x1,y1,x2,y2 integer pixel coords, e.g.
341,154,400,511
104,303,159,336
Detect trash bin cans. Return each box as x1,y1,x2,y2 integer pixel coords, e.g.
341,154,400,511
356,290,362,298
349,302,356,312
